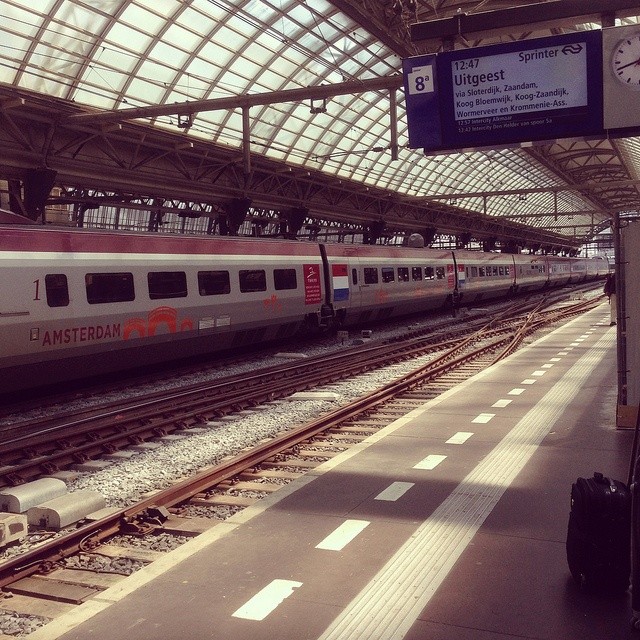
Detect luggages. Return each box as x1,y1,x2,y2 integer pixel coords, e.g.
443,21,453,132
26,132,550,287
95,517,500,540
566,406,639,592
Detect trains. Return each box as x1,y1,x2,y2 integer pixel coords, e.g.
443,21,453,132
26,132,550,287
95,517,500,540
0,225,614,394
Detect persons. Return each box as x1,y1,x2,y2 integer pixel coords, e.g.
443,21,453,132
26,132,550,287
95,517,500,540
603,272,617,326
